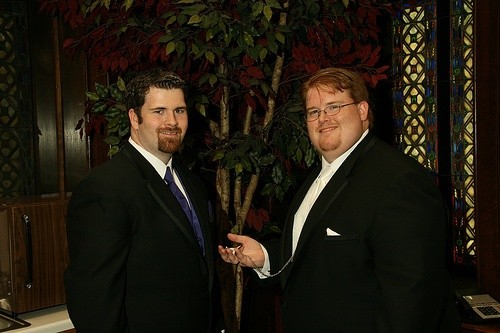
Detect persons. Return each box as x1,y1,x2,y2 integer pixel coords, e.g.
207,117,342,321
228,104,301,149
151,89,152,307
218,68,444,333
63,67,226,333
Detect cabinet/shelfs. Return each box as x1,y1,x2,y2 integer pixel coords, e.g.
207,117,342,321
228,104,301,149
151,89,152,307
0,196,70,318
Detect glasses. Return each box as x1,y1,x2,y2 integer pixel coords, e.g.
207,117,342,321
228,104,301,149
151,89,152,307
303,100,363,122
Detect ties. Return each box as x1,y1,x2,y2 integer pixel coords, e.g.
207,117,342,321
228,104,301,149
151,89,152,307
293,165,334,252
164,167,193,226
